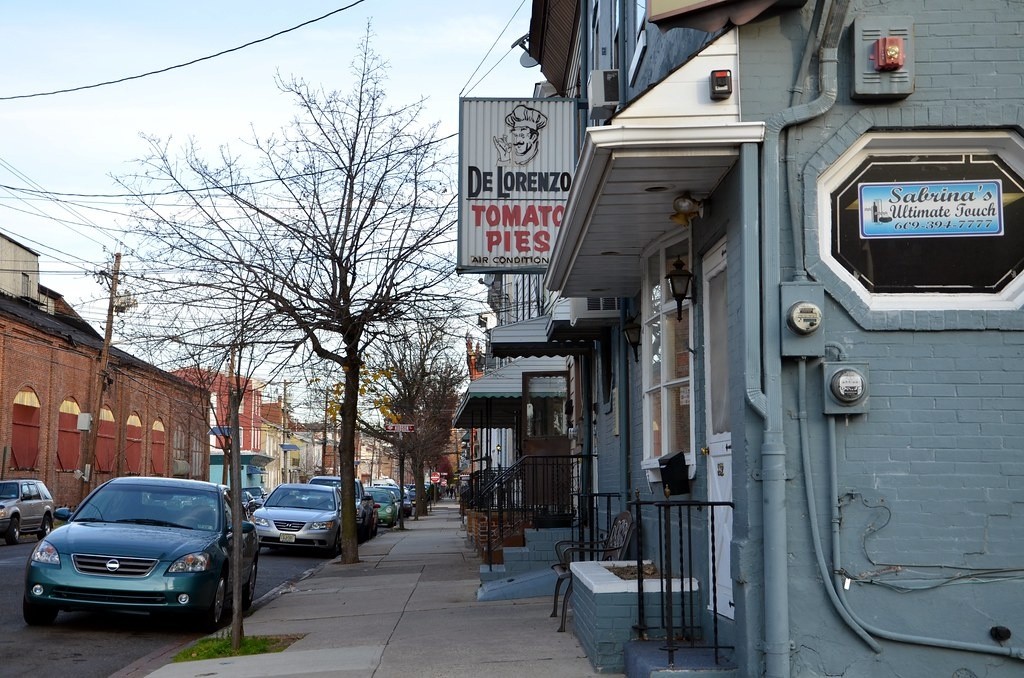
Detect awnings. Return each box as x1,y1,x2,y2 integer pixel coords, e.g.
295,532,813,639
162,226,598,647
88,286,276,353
452,355,567,429
490,313,596,358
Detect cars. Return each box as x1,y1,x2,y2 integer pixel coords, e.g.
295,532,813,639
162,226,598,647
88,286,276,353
250,484,342,559
241,486,268,521
23,477,261,634
364,475,431,537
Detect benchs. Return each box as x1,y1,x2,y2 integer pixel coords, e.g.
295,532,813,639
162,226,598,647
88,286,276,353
139,503,188,522
297,497,319,507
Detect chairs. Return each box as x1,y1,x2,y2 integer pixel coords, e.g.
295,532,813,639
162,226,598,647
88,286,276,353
551,510,634,632
318,497,330,508
113,494,139,519
183,503,216,530
278,494,296,506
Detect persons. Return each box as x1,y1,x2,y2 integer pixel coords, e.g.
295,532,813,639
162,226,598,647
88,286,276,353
446,486,454,499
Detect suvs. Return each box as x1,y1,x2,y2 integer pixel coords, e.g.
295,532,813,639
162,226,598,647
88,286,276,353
310,476,372,543
0,478,55,545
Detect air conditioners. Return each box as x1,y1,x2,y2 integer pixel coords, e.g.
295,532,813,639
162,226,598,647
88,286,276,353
587,69,619,119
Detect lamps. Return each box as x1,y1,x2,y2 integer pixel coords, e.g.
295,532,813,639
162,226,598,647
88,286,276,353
496,443,501,455
664,253,697,322
621,309,642,364
669,193,710,226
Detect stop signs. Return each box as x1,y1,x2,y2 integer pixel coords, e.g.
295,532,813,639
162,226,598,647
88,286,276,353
431,472,440,482
